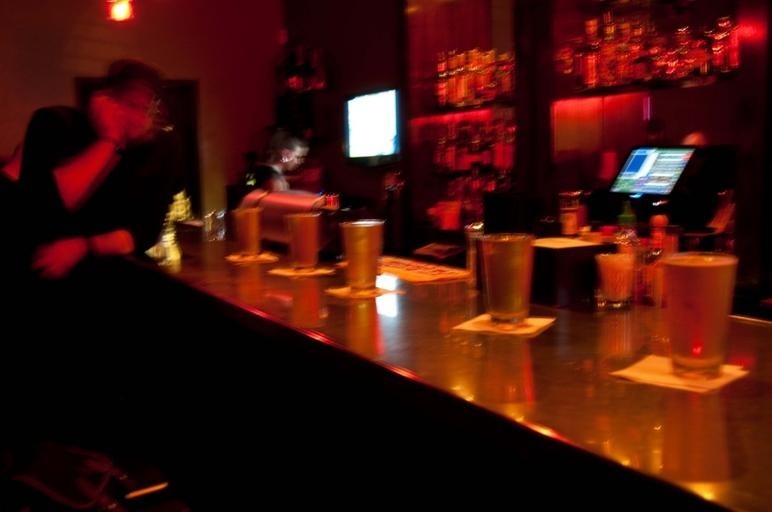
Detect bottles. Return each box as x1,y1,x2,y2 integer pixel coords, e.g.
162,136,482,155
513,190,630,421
617,200,645,306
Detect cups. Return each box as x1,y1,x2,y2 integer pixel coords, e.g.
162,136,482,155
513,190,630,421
478,232,536,327
282,209,324,271
658,251,742,380
599,251,633,304
232,205,264,259
340,218,388,296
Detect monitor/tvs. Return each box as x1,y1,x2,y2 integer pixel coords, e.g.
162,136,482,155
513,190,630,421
345,86,405,163
601,144,699,210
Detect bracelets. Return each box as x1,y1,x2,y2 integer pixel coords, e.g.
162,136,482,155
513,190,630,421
85,235,96,263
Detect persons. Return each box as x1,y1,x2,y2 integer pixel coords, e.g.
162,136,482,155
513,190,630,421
228,135,315,256
0,55,186,510
